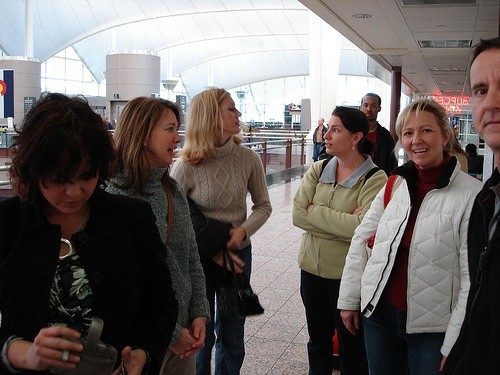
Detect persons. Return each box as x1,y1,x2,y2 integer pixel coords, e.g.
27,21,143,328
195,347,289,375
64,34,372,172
100,97,210,375
103,117,117,130
0,91,179,375
170,87,272,375
292,37,500,375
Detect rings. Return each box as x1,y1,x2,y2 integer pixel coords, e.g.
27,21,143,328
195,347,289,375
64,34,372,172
63,351,70,362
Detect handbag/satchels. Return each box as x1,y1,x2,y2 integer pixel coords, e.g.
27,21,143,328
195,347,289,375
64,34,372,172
222,245,264,316
